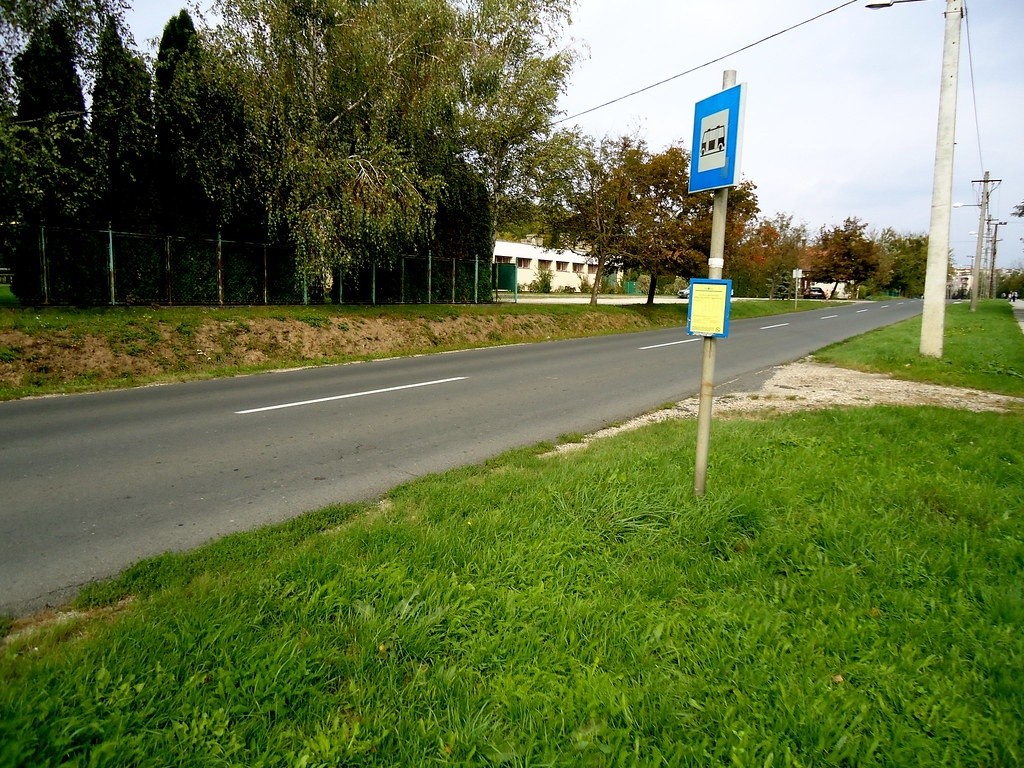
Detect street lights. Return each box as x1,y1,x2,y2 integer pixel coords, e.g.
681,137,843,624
952,203,986,312
968,230,990,300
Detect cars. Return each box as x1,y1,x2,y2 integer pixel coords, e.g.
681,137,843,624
678,283,691,299
803,286,826,299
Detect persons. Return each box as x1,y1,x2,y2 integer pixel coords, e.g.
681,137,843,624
1008,290,1017,302
